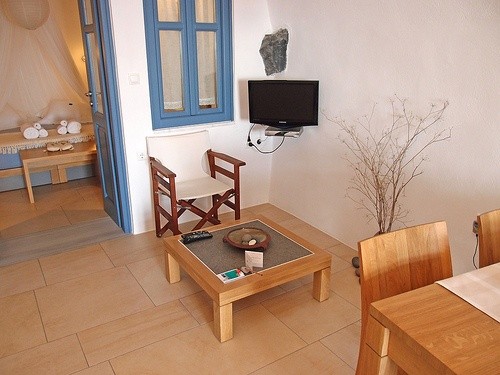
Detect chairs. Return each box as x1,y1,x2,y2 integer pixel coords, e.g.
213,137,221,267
146,130,246,239
477,209,500,269
356,221,454,375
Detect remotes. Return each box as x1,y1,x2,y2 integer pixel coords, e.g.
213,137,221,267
181,230,213,242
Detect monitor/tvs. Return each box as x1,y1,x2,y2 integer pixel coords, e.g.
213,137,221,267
248,80,319,130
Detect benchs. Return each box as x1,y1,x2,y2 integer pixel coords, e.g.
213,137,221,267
19,142,98,204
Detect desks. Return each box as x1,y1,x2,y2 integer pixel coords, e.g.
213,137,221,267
163,214,332,343
361,262,500,375
0,122,98,184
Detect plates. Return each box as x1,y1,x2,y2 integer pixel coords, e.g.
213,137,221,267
223,227,271,250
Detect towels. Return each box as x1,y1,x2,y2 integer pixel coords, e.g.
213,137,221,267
57,120,82,135
20,122,48,140
